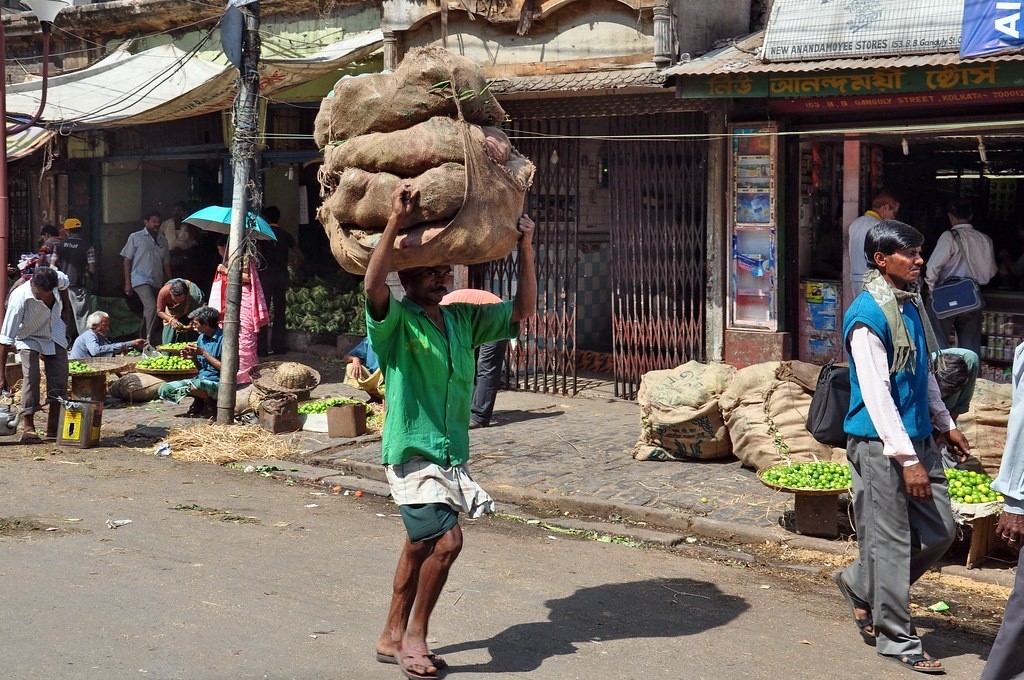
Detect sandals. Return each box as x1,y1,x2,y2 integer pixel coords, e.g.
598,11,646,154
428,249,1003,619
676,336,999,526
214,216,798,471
877,651,944,672
835,570,877,638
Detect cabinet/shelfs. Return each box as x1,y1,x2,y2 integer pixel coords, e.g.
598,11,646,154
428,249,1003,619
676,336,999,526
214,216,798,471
941,290,1024,386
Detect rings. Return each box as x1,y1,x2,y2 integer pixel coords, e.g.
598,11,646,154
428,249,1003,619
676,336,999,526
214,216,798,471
1002,533,1009,538
1009,537,1016,542
126,292,128,295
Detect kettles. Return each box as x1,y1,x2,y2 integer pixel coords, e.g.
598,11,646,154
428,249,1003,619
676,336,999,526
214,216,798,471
0,403,21,435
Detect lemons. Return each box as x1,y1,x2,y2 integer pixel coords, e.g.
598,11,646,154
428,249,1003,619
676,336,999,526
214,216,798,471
761,461,854,490
942,468,1005,504
136,341,197,369
297,399,368,413
68,360,93,373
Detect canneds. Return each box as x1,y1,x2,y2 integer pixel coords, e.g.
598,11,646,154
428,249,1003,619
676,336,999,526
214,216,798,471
981,312,1024,360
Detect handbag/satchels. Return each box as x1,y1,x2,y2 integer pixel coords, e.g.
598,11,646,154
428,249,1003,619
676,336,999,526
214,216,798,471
930,275,985,321
806,365,851,449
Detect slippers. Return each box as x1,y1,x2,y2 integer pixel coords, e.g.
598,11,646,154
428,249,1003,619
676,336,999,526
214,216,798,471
19,437,44,444
393,646,439,680
376,650,448,667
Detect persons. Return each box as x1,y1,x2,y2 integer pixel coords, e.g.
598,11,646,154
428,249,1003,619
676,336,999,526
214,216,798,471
849,193,899,299
34,219,95,336
343,336,386,405
182,206,277,280
365,183,537,680
835,220,971,671
980,341,1024,680
924,198,997,378
0,266,70,443
71,311,150,360
158,307,223,417
999,223,1024,291
251,207,304,354
217,233,270,384
438,288,508,429
931,347,979,469
120,202,207,355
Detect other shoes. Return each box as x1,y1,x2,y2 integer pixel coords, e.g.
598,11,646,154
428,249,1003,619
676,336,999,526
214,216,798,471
187,396,217,419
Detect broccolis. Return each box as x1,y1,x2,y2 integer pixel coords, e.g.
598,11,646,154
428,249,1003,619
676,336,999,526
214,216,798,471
285,281,368,334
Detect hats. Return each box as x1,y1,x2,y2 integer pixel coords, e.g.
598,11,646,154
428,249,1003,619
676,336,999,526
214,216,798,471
40,225,58,235
59,218,81,231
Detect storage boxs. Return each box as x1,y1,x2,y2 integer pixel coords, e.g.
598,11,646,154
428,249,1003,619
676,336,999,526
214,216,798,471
298,401,365,438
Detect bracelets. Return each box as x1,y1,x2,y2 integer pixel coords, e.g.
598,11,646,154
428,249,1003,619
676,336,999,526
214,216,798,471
168,317,175,324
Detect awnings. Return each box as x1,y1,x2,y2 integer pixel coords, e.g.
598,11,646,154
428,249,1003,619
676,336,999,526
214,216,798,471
5,10,384,163
